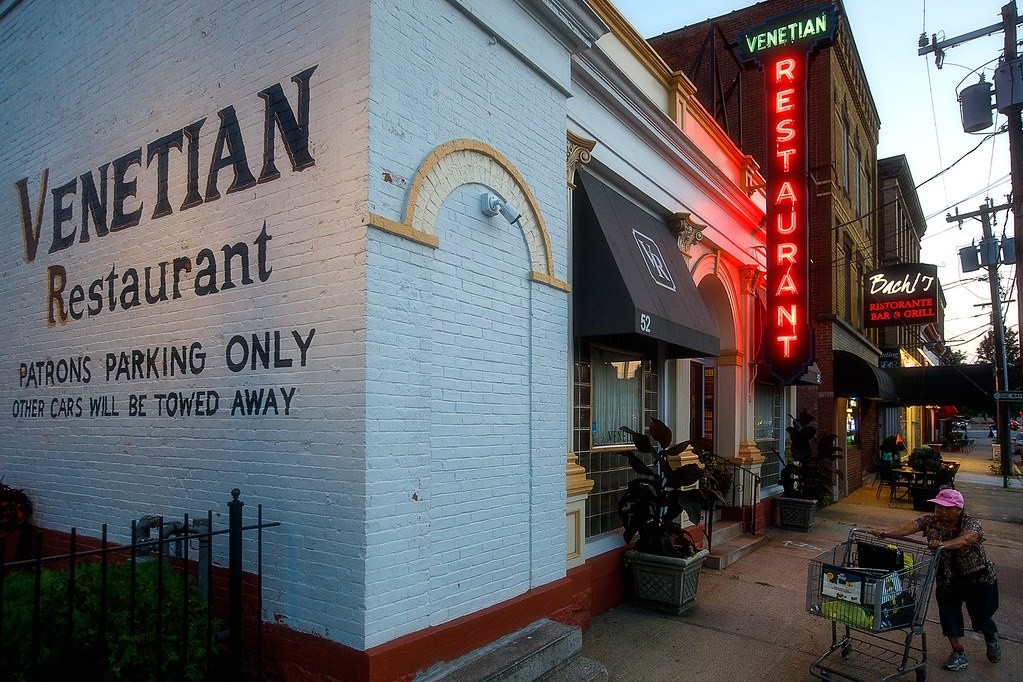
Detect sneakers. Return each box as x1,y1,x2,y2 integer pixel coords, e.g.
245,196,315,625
984,633,1001,663
943,651,969,670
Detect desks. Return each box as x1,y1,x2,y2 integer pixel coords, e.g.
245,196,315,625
892,463,951,500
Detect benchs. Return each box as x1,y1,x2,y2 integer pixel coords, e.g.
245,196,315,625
960,438,975,453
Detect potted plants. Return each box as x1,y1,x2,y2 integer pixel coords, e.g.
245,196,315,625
907,444,944,511
878,435,905,481
773,409,843,532
618,415,710,615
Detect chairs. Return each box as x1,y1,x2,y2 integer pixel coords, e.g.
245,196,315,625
872,459,960,504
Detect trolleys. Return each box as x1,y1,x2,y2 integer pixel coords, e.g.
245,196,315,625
807,528,947,682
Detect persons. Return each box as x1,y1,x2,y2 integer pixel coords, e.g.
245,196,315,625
871,489,1002,671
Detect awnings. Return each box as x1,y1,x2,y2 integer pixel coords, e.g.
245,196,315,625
573,170,720,357
834,349,897,397
941,405,959,416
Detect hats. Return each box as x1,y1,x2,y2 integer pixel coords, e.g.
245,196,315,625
927,489,964,509
